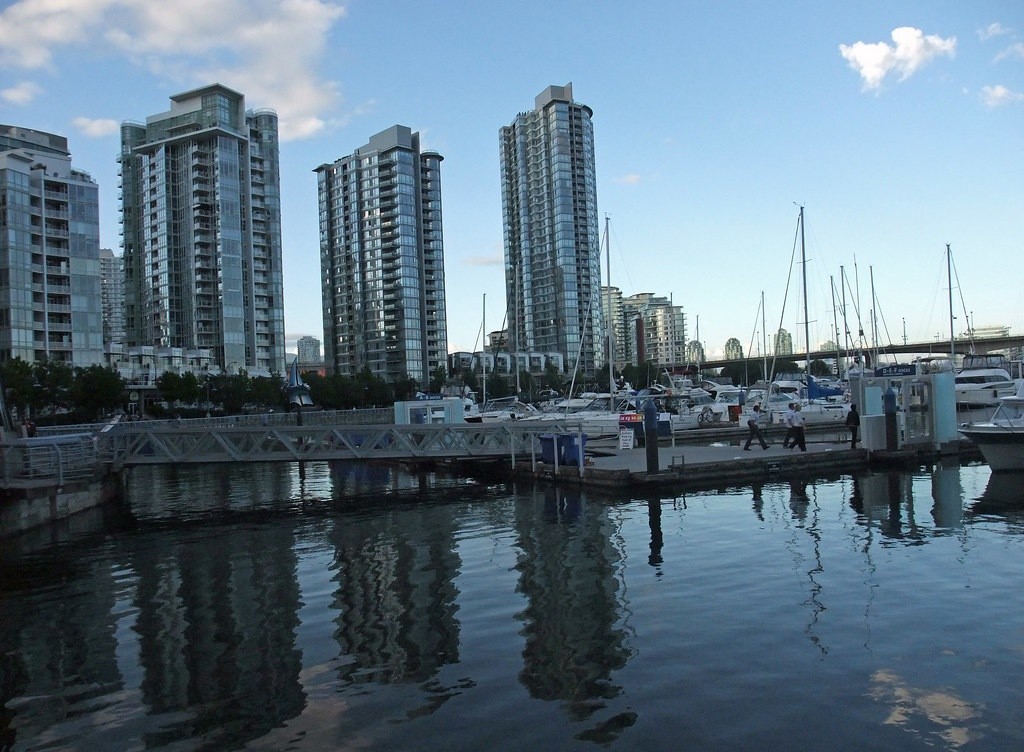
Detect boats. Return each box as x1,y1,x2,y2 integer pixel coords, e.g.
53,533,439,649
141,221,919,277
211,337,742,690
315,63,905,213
956,384,1024,474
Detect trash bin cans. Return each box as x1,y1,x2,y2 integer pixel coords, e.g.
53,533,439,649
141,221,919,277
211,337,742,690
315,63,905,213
560,432,587,466
536,435,562,464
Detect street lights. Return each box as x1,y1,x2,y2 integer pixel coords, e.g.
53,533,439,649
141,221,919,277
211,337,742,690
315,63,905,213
205,377,211,417
361,387,368,410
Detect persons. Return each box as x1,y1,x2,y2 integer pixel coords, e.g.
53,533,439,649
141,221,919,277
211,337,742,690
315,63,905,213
26,418,36,438
844,403,860,449
783,402,808,451
743,404,771,451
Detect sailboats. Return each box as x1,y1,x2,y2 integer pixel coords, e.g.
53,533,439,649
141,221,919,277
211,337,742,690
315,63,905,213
423,211,1024,454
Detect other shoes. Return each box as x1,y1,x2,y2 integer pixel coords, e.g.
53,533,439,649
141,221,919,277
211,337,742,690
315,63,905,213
801,450,809,454
851,446,857,449
763,446,771,450
783,446,789,449
788,446,794,453
744,448,752,451
